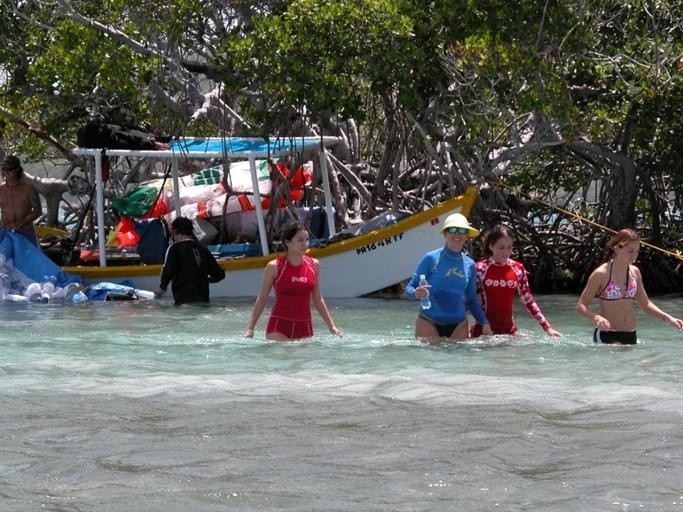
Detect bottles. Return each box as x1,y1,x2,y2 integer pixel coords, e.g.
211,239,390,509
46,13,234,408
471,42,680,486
418,273,432,311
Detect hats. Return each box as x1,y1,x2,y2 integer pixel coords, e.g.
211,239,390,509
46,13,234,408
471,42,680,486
439,213,480,238
1,155,20,170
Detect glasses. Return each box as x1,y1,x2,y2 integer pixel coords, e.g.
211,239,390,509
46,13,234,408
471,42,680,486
446,227,468,235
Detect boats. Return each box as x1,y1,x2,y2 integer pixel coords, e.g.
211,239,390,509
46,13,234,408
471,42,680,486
51,130,479,300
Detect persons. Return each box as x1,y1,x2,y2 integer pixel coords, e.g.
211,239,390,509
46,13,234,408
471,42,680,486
576,228,683,345
404,214,493,346
245,221,343,345
260,146,323,209
0,155,42,248
470,225,563,339
154,217,225,307
